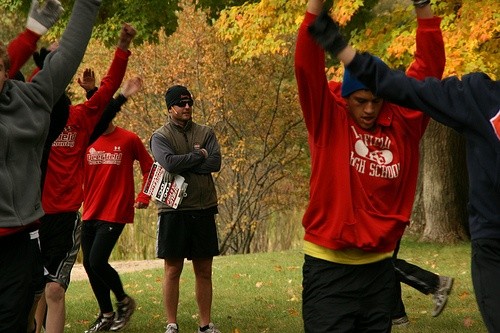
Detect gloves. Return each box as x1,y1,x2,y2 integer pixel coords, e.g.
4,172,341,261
308,12,348,58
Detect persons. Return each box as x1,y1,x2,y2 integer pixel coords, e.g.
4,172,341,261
149,86,223,333
308,10,500,333
391,257,455,327
80,121,155,333
0,0,143,333
293,0,446,333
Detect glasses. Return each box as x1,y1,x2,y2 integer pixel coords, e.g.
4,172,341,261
174,101,193,107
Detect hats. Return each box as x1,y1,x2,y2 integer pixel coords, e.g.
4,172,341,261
341,56,386,97
165,86,192,110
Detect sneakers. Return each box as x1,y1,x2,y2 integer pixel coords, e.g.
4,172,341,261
110,294,136,331
432,275,454,317
389,314,409,326
85,312,116,333
165,323,178,333
199,323,218,333
